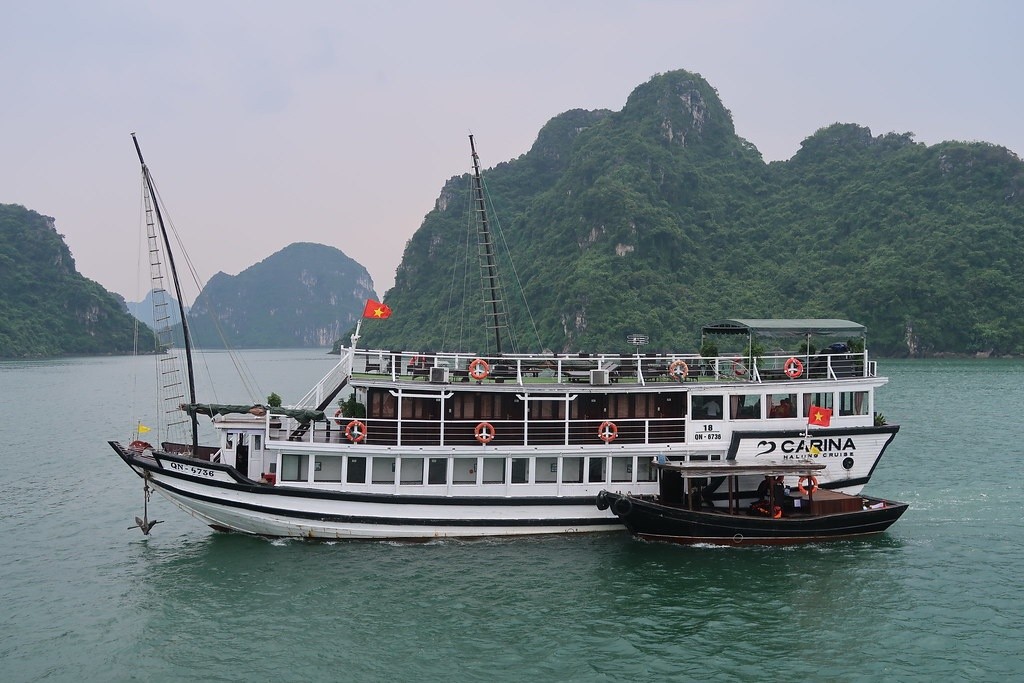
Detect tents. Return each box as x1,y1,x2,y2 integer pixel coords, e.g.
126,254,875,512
701,319,868,380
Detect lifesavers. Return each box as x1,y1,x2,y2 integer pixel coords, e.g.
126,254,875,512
783,358,804,378
798,476,819,496
597,421,618,442
334,409,342,425
614,497,634,518
409,356,427,365
669,359,689,381
345,420,366,443
468,359,489,379
474,422,495,443
732,359,747,375
596,490,609,510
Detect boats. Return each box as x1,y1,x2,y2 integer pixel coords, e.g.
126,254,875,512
105,132,901,544
594,459,911,545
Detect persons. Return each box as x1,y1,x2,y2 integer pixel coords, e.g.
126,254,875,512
775,398,796,418
756,475,784,507
702,400,720,420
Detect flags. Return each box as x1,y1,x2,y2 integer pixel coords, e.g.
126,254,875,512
808,406,831,427
363,299,392,320
